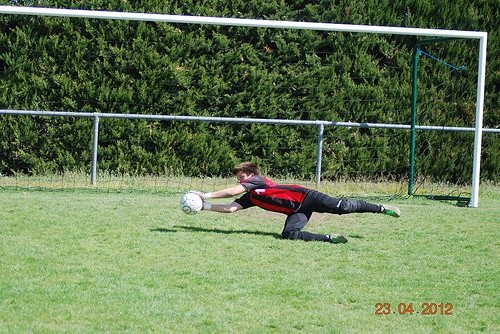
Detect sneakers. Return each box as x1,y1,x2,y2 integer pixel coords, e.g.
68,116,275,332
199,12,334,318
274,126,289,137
379,203,400,218
330,234,348,243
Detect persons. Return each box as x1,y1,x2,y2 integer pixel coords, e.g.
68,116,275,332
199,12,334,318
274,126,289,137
188,162,400,244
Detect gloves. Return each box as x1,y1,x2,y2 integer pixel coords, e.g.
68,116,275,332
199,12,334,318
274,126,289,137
201,199,212,211
188,191,211,200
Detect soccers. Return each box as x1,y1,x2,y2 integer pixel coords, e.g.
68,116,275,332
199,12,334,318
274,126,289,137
180,193,203,215
